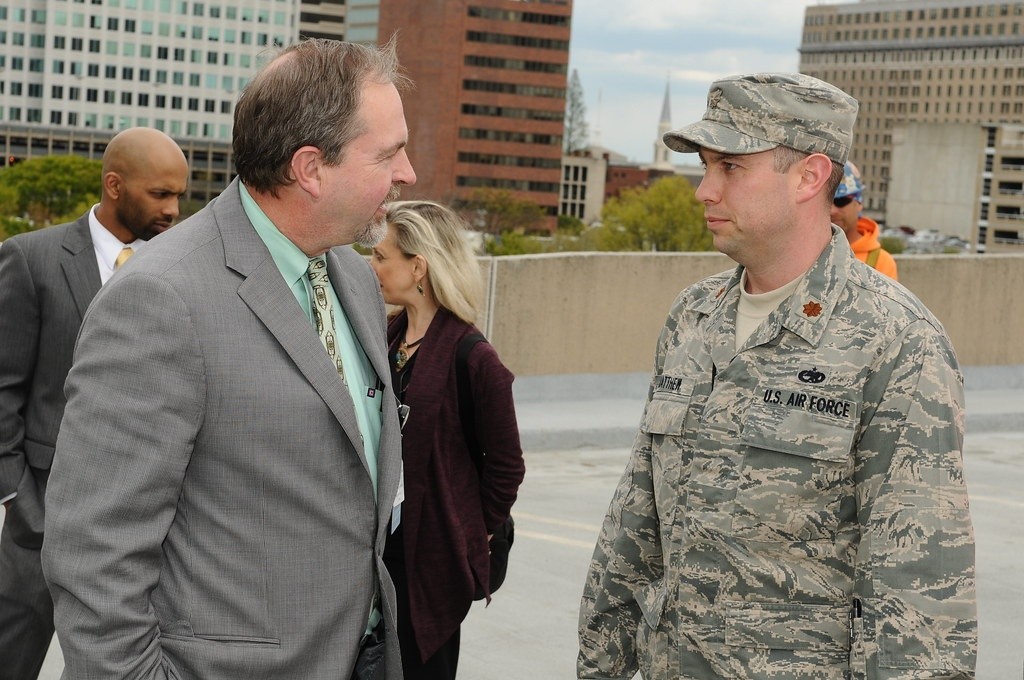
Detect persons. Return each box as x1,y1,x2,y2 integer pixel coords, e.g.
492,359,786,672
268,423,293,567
41,38,416,680
830,161,898,283
370,201,525,680
578,71,979,680
1,127,189,680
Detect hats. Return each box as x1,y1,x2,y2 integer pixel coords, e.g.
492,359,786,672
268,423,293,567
663,73,859,166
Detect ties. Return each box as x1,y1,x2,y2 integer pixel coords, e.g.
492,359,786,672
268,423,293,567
118,249,133,267
307,252,349,394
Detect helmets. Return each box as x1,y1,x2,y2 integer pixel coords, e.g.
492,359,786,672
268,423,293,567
834,161,862,198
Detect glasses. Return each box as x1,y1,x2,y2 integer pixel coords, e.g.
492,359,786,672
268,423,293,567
834,194,856,207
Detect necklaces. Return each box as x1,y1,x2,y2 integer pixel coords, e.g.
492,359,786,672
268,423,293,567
396,333,422,368
